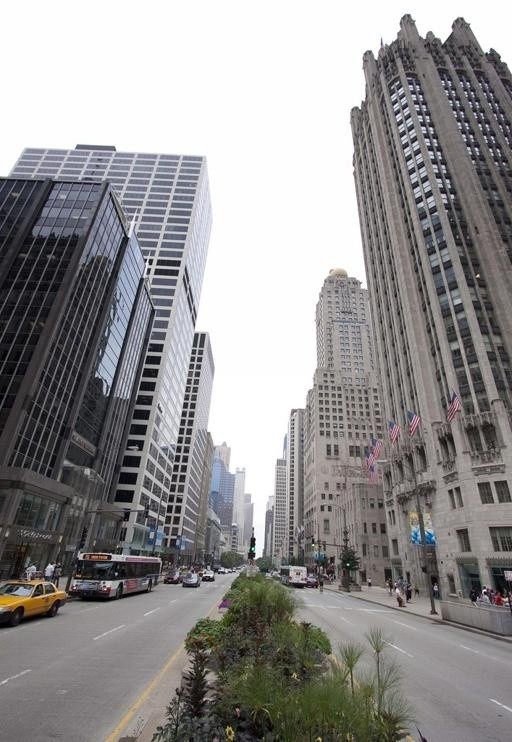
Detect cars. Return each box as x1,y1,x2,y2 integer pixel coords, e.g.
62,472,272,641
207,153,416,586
164,567,236,587
0,580,68,626
266,566,319,588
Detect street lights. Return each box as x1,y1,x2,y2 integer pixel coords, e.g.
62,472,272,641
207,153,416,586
312,503,350,577
151,495,184,556
376,460,439,614
65,445,150,594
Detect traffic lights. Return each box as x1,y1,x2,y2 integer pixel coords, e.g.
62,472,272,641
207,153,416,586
312,538,315,547
250,537,256,553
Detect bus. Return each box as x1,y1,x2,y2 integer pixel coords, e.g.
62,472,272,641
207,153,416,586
69,552,162,600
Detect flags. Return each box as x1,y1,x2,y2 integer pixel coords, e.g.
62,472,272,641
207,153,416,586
407,411,421,438
365,438,384,482
448,391,460,423
388,421,401,447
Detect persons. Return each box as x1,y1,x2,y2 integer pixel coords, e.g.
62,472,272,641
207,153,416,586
386,576,418,607
433,583,439,598
330,575,336,583
25,562,36,581
469,585,512,606
367,577,372,587
44,561,63,589
319,575,324,592
